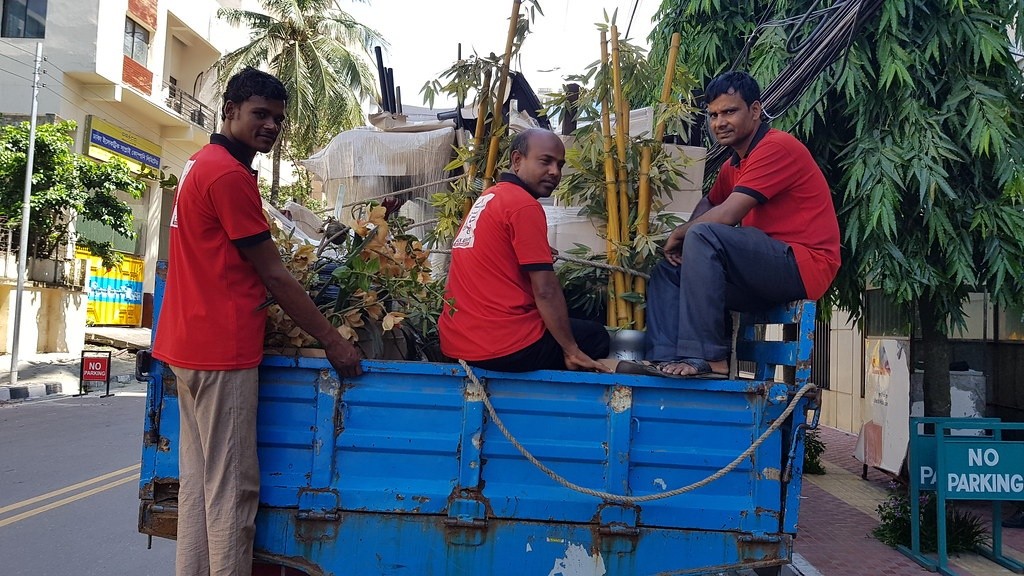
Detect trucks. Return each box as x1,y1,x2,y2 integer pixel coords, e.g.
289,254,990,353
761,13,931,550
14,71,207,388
134,261,816,574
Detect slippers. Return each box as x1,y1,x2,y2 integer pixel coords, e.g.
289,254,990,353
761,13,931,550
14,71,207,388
658,357,729,380
615,358,659,373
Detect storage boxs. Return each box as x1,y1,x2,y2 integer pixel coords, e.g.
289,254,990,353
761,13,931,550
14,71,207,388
262,319,408,361
650,190,703,213
649,144,708,191
536,135,707,253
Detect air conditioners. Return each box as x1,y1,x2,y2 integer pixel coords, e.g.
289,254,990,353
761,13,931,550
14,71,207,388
189,108,208,129
168,98,184,115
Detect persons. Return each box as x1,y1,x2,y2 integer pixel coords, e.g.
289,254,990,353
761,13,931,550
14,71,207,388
617,71,841,379
152,66,363,576
437,129,614,373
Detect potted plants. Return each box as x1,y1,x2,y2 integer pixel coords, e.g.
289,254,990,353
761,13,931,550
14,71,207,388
551,8,706,360
